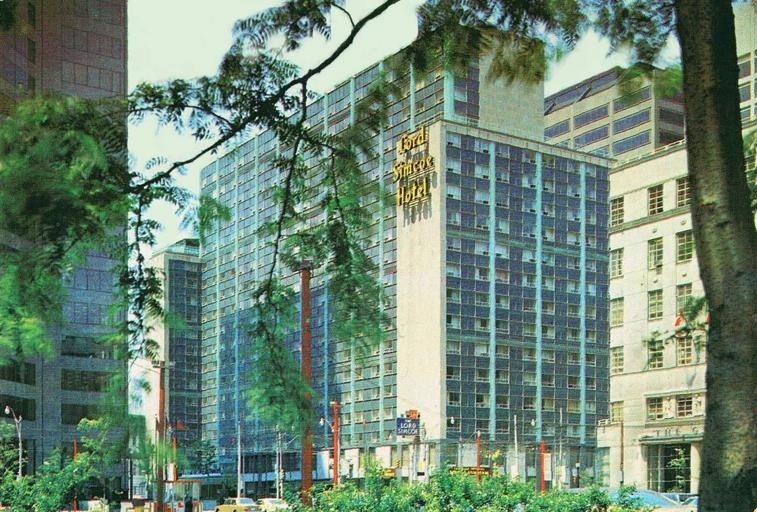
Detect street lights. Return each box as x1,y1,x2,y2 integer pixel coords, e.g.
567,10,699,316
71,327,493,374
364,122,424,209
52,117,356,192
319,417,341,484
3,406,24,480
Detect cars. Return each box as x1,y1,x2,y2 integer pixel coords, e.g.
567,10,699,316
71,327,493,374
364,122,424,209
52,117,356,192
567,485,700,511
256,498,291,511
215,496,263,512
